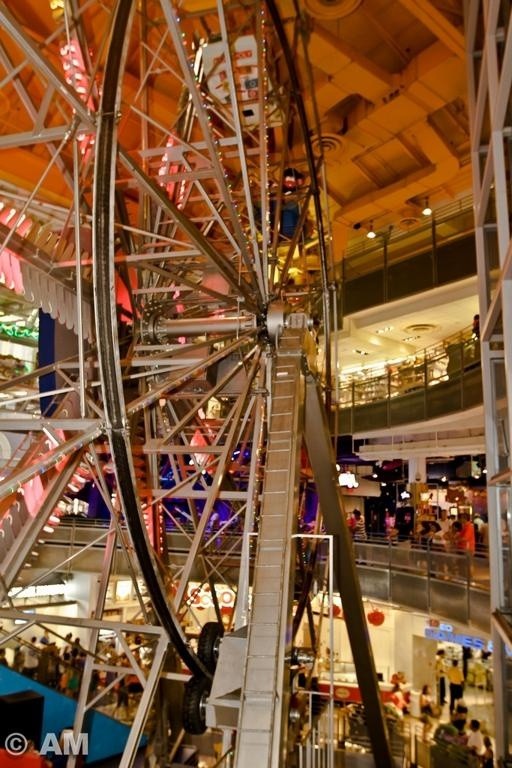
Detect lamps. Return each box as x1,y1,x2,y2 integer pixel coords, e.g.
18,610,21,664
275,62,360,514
363,215,381,240
417,193,436,221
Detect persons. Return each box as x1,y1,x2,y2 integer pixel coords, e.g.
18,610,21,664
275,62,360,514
3,308,511,768
